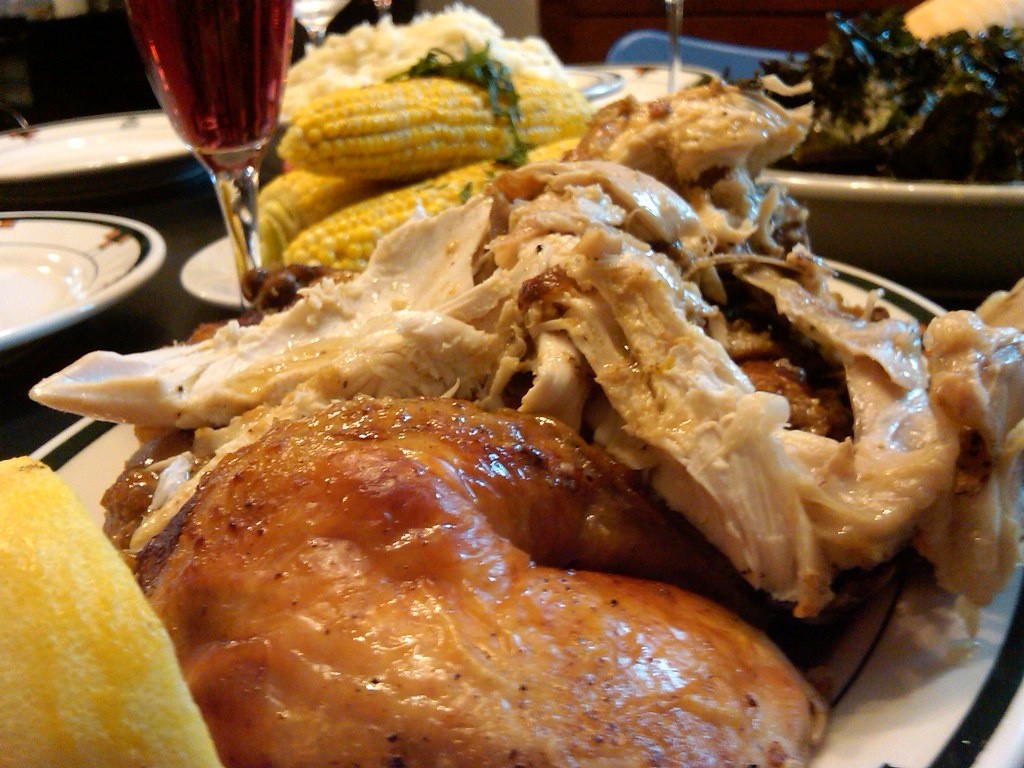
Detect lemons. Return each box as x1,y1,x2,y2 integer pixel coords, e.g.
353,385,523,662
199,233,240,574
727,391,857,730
0,456,220,767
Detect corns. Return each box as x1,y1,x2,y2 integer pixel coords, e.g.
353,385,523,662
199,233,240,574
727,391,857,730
259,74,597,270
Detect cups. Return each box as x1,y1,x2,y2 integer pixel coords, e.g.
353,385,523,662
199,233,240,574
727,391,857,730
293,0,353,43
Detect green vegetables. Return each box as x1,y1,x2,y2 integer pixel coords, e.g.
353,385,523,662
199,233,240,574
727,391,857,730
384,37,538,168
718,0,1024,186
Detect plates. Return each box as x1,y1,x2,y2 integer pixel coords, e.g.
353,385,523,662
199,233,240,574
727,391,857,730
742,162,1024,279
179,228,269,311
27,257,1024,768
1,211,168,357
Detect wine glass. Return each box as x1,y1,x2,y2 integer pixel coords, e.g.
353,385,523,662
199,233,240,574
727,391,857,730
125,0,294,324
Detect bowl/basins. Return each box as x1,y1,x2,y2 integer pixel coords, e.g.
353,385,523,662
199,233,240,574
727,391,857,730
0,112,212,210
